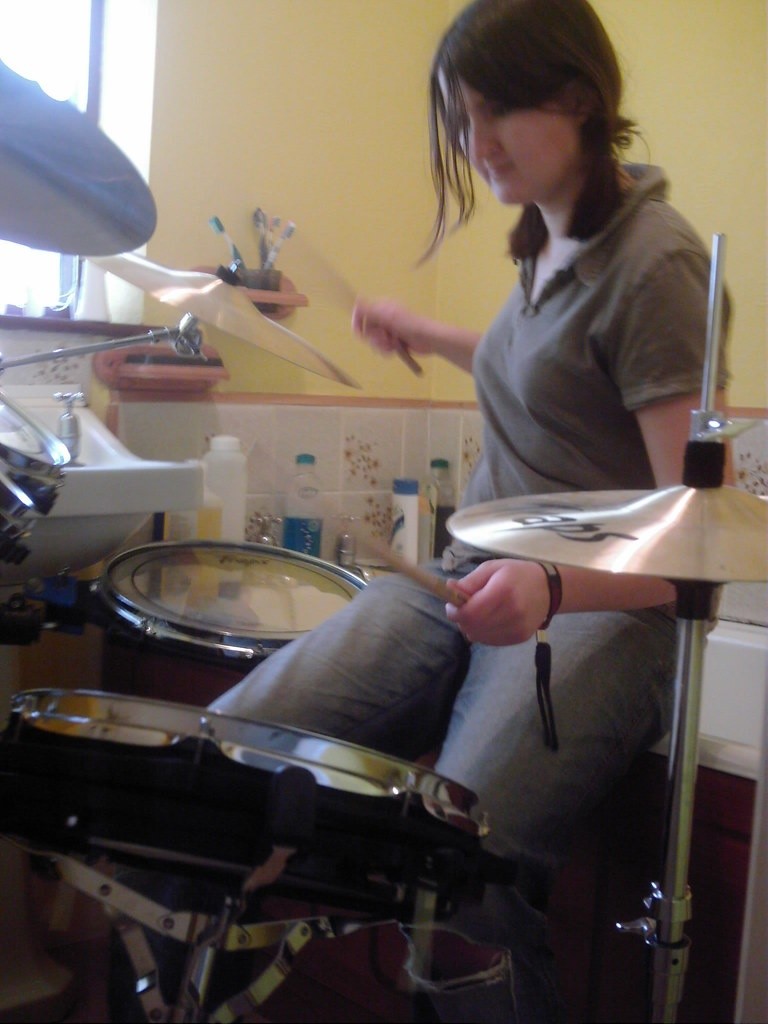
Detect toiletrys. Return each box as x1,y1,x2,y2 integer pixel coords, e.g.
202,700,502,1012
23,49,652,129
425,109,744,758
389,478,419,570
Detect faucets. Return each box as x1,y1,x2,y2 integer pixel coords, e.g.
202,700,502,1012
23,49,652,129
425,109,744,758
43,390,86,462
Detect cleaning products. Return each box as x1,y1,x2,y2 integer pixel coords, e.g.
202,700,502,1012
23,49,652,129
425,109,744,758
202,433,249,545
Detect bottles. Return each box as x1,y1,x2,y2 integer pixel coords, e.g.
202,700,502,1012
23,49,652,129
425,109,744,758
426,458,455,559
282,453,323,559
195,435,247,544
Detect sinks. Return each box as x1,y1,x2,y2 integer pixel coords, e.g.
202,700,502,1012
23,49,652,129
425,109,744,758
0,382,205,587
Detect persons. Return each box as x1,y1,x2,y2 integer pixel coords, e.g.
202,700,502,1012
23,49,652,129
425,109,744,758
107,1,735,1024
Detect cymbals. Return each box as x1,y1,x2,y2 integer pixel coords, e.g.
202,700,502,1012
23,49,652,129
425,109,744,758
88,250,365,393
0,56,158,259
445,484,768,583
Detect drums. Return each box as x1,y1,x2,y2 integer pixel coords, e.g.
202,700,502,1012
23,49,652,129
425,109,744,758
98,538,369,709
2,680,482,914
0,392,73,544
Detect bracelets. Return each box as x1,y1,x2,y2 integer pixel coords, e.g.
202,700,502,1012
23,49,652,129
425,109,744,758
535,562,562,751
